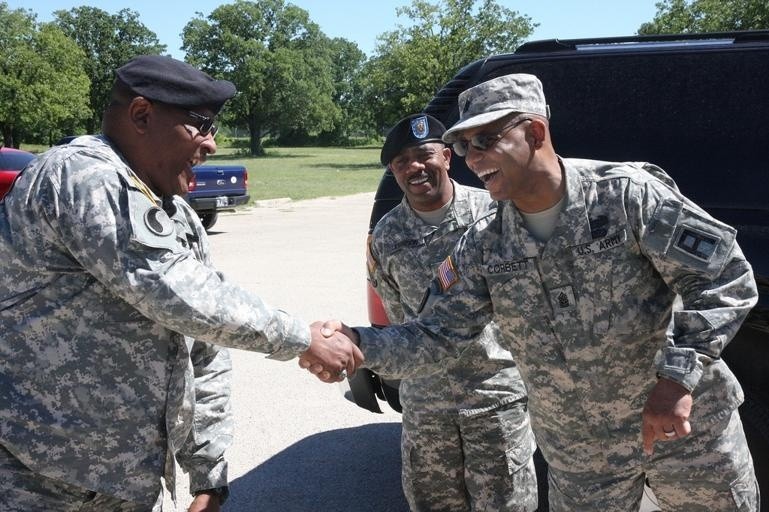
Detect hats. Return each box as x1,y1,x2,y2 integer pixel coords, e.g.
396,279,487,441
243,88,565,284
381,114,446,166
442,73,550,143
116,56,235,107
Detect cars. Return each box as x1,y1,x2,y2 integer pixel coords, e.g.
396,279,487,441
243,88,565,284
0,146,39,197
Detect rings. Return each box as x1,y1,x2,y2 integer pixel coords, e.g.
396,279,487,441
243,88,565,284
337,369,348,378
663,430,675,437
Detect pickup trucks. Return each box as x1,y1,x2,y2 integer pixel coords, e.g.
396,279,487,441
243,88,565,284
189,166,251,229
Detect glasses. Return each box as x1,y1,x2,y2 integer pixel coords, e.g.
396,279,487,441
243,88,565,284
183,111,219,138
452,118,529,157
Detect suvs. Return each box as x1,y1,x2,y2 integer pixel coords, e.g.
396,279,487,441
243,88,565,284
344,29,769,414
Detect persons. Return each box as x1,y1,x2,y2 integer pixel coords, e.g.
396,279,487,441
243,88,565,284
0,56,364,512
299,73,761,512
370,113,539,512
